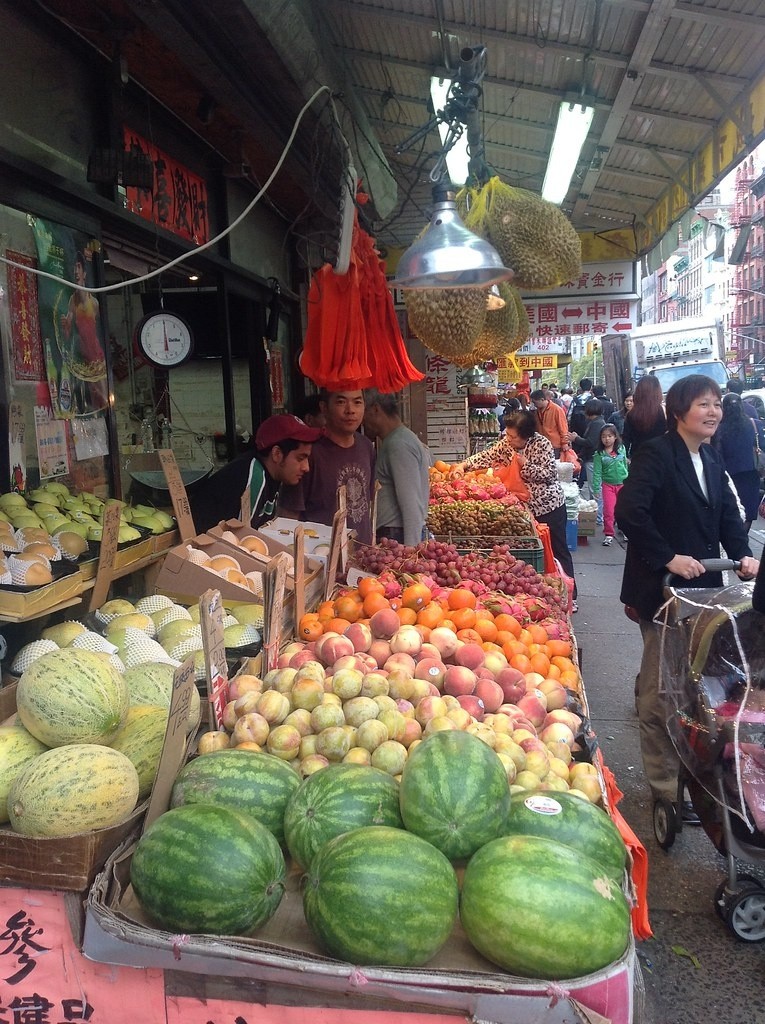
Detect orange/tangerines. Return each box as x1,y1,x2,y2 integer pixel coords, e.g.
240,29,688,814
426,460,501,484
472,613,580,688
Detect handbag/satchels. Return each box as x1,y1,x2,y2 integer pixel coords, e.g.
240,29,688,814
753,447,765,478
559,450,582,474
491,454,531,502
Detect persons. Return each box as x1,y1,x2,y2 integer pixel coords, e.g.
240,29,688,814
363,387,431,547
711,393,765,534
716,675,765,776
614,374,762,823
143,414,331,597
62,251,108,410
279,388,376,553
446,409,579,614
622,375,669,464
497,375,759,491
593,423,628,546
565,399,606,512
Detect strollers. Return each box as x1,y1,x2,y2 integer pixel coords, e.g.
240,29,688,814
652,558,765,943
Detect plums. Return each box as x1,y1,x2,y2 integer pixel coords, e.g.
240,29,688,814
197,660,602,808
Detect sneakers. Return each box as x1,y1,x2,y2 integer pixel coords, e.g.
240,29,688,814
572,476,580,482
602,535,615,546
621,532,629,541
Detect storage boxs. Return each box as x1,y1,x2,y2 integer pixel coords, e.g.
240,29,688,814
566,520,578,551
1,517,359,892
0,497,181,618
578,510,597,537
430,536,545,573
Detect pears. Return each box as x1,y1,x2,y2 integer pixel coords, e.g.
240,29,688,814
15,593,267,674
0,482,173,585
180,530,295,602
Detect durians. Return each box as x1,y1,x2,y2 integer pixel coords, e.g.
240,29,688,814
401,192,582,369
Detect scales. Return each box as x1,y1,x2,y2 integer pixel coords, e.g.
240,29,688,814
121,294,214,492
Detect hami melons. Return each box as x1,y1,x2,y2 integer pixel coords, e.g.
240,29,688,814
0,648,201,841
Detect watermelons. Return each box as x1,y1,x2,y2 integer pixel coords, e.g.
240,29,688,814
129,727,631,982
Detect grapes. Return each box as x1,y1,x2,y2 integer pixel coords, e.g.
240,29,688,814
523,562,570,611
335,537,523,593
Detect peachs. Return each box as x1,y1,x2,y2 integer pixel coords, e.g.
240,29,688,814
272,609,586,751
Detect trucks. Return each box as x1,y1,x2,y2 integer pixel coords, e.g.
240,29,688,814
601,311,737,424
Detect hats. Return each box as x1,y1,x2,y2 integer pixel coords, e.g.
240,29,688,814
254,413,321,451
504,397,521,411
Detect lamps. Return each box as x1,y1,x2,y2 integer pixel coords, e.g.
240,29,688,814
541,91,595,205
458,365,495,388
428,68,474,186
486,284,506,311
384,46,515,292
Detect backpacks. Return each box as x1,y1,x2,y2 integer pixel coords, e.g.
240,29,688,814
569,394,595,437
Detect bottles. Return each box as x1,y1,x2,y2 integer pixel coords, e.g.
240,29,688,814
141,419,154,454
161,418,173,449
44,338,60,420
39,428,63,456
59,342,74,419
